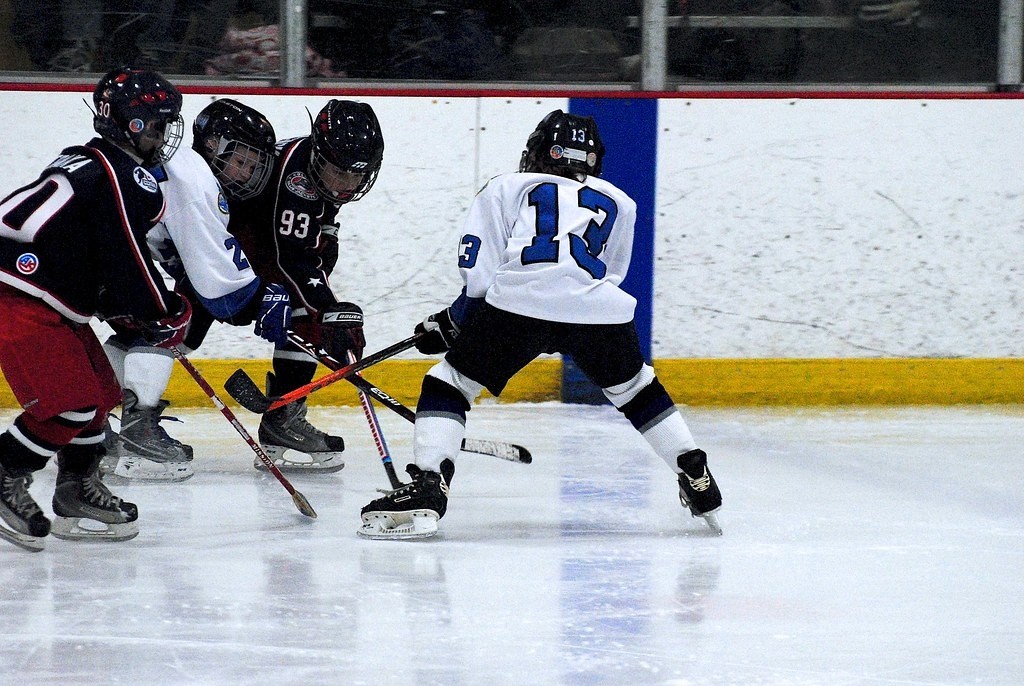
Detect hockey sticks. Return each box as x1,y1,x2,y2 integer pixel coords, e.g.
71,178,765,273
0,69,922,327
223,331,423,416
167,345,318,520
342,350,412,488
285,332,534,466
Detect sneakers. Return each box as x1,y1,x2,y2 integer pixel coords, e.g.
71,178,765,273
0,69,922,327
50,445,139,543
254,371,346,474
98,388,195,484
0,457,51,553
677,448,723,536
356,458,455,540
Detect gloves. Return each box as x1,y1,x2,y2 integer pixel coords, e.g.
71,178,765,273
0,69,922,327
254,282,292,349
414,307,462,355
316,222,340,277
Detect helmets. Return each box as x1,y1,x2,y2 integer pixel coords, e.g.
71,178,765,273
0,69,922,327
93,65,182,146
526,109,605,177
193,98,276,154
311,99,385,176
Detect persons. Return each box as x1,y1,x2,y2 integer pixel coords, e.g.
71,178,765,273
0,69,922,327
0,1,998,81
356,109,723,539
56,99,384,482
0,65,195,552
